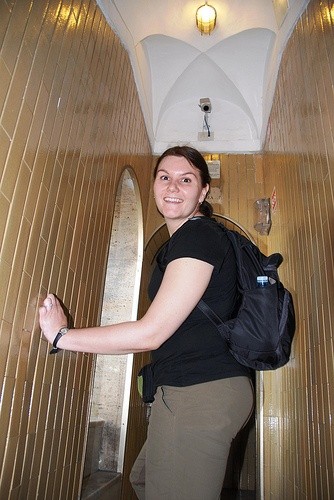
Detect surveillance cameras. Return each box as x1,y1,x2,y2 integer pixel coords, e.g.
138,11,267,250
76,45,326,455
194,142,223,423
200,98,211,113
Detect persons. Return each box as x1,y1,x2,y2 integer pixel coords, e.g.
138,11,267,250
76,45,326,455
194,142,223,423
39,146,255,500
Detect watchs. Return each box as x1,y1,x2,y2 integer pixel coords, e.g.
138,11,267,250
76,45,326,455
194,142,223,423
49,327,69,355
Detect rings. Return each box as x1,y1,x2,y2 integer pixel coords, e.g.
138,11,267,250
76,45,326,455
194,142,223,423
46,304,53,308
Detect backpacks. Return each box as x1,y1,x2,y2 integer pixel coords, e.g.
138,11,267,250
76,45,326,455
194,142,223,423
151,216,295,371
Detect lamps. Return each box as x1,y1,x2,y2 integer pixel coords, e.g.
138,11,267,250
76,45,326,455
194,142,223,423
195,1,217,37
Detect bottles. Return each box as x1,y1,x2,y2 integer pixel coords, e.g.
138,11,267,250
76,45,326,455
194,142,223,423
256,277,268,288
